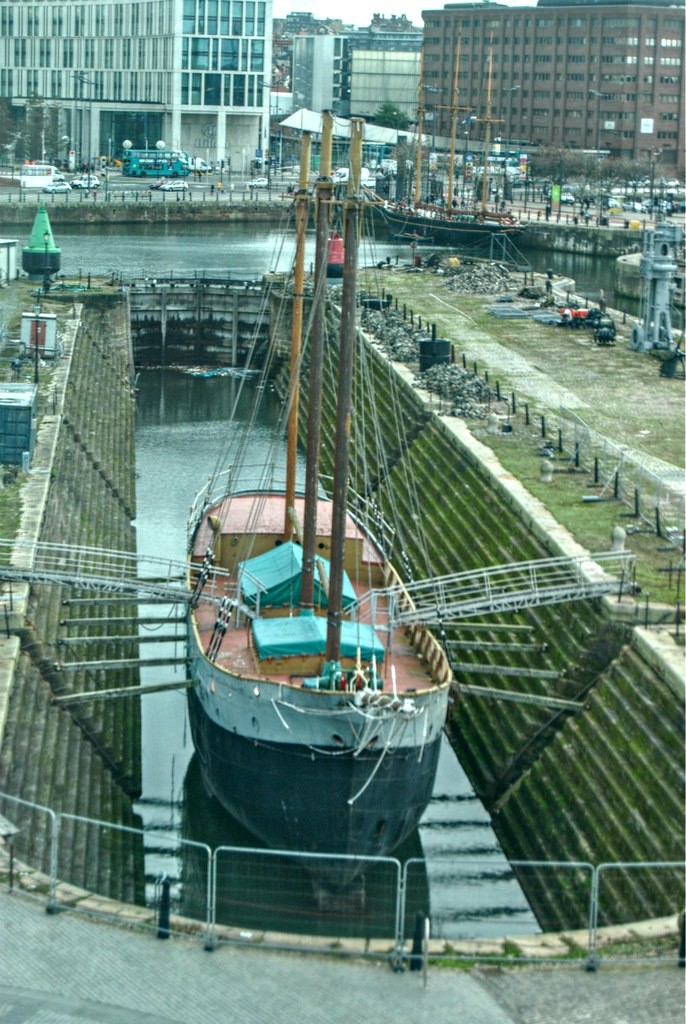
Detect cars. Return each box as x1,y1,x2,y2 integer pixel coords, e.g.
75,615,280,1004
248,177,268,188
360,178,376,188
149,178,165,189
161,179,188,193
43,183,72,194
520,176,686,223
69,175,100,189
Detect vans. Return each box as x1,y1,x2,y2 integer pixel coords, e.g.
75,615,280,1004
332,167,348,183
188,156,212,173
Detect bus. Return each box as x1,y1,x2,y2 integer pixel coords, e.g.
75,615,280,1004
464,149,526,188
22,164,65,182
123,149,190,177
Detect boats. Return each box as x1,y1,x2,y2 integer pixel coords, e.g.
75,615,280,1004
369,20,527,247
191,110,455,892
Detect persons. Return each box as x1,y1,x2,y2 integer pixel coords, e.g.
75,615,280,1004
392,184,682,228
286,183,295,196
22,158,225,192
